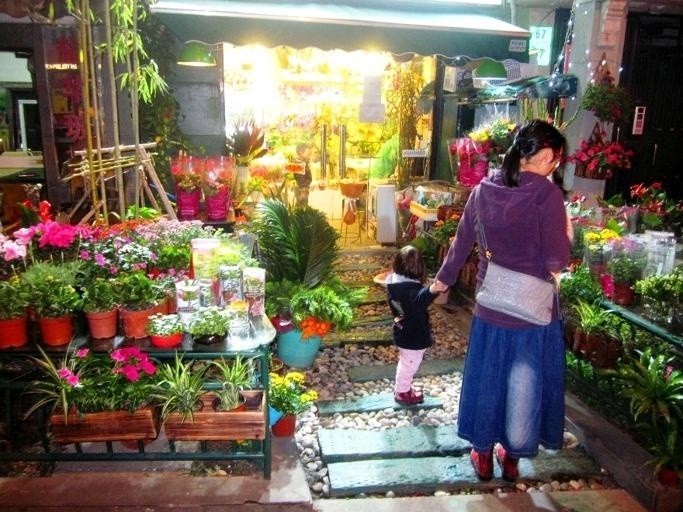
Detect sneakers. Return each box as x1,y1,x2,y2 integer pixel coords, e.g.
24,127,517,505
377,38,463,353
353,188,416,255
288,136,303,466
394,388,424,404
411,385,423,393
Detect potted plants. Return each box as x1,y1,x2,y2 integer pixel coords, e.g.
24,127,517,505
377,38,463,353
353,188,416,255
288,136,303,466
149,348,266,427
225,112,268,182
0,260,267,349
557,252,683,512
255,198,369,368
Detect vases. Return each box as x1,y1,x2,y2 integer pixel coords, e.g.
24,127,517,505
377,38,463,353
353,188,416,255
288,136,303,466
574,164,612,180
271,414,296,437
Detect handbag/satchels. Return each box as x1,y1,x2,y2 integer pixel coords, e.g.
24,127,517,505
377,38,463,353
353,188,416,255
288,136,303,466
474,261,554,327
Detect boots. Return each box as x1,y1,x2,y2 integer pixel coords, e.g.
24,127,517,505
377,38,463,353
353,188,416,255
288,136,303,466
470,448,493,481
496,447,519,482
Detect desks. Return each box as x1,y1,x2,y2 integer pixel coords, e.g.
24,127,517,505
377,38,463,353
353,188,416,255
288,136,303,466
203,217,235,233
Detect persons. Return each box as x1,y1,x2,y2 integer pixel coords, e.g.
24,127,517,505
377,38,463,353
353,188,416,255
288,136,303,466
292,143,312,207
435,117,573,479
371,126,416,217
382,245,443,403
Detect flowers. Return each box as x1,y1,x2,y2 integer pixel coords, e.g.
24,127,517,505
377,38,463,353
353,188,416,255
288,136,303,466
564,184,683,252
22,336,157,426
268,371,319,415
445,120,517,186
168,156,236,220
0,200,204,299
566,140,635,175
